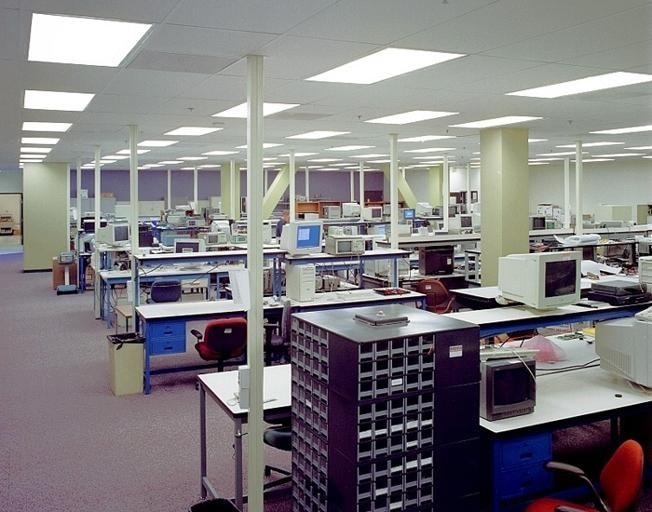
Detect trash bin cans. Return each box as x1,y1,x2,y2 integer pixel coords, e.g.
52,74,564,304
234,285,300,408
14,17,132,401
107,333,146,396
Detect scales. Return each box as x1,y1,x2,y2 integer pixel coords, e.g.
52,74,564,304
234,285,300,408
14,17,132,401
57,252,78,295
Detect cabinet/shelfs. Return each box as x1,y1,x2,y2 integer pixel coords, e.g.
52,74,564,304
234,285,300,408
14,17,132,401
297,200,341,218
